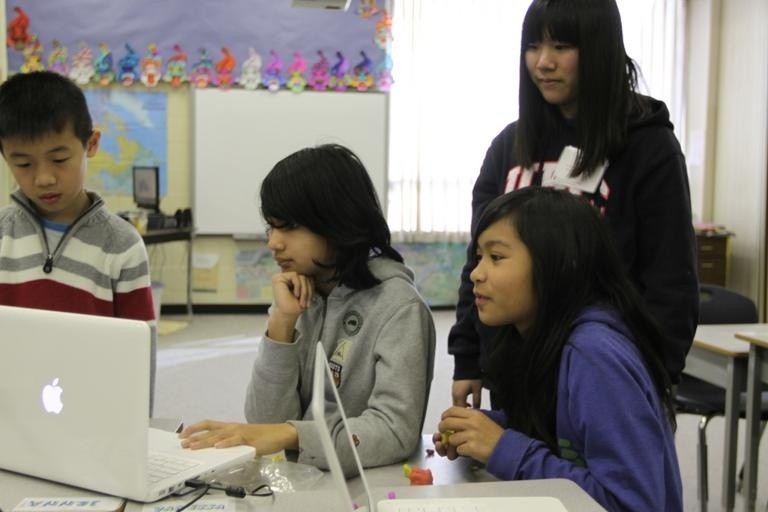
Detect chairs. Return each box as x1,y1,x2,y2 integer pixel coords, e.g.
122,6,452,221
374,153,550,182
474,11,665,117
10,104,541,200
668,285,758,510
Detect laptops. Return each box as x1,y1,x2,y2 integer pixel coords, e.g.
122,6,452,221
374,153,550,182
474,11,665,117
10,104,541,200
310,340,567,512
0,304,257,503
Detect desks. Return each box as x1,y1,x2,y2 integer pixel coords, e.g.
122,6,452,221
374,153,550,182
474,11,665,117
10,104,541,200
137,229,194,321
0,416,608,511
688,321,768,512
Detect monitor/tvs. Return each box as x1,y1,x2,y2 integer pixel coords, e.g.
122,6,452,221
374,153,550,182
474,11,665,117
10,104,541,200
133,166,159,209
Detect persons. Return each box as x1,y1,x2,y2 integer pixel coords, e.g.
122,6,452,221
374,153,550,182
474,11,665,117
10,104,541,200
432,184,685,512
2,70,160,420
444,2,700,437
174,141,439,480
